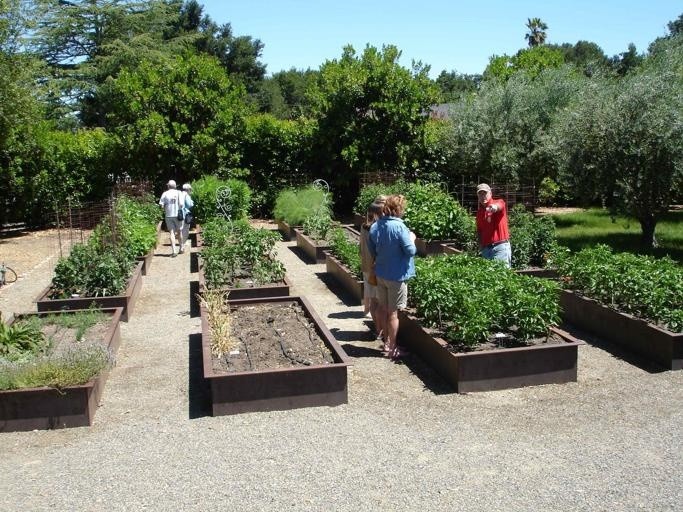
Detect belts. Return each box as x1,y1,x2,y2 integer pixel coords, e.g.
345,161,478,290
487,240,508,249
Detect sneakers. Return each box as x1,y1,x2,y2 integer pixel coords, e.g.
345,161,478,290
172,249,185,257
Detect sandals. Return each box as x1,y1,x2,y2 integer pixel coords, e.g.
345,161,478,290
375,329,411,358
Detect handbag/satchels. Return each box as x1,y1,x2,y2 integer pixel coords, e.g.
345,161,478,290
186,213,195,224
178,209,183,221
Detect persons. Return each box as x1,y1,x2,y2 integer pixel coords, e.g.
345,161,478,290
476,183,512,270
179,184,194,247
367,195,417,358
359,195,387,340
159,180,185,258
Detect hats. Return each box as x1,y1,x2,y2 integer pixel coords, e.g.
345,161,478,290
476,183,491,194
183,183,193,190
165,180,177,188
